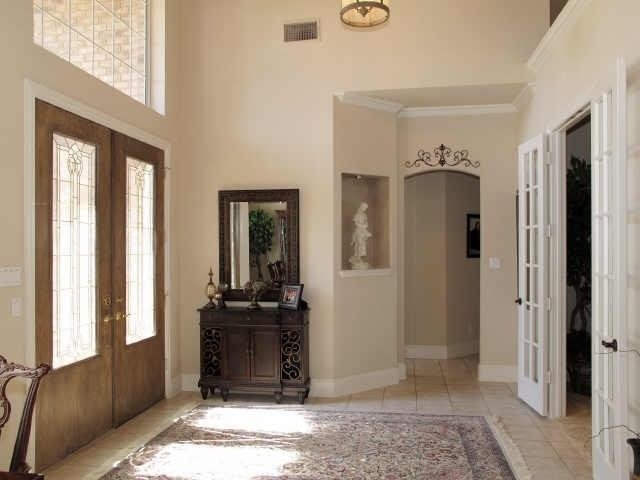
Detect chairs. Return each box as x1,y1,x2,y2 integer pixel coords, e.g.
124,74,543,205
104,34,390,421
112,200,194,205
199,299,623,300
0,355,50,473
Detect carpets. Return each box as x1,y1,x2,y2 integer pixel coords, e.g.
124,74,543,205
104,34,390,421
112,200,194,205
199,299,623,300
85,403,533,480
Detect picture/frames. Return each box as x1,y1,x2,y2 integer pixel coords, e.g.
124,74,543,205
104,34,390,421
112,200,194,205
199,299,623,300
277,283,303,311
466,213,480,259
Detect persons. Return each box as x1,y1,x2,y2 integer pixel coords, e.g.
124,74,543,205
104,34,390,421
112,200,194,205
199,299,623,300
352,201,372,260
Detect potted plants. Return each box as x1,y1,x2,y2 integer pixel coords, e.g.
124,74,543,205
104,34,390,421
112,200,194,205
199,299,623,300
249,208,275,291
566,156,591,397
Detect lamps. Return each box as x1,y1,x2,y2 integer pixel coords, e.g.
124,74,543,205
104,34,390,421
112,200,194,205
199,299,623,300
339,0,390,28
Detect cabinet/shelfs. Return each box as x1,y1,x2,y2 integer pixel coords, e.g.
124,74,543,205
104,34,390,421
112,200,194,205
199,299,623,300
197,306,309,405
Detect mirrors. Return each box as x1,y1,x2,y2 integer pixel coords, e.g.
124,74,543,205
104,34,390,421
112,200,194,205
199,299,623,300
217,189,300,302
